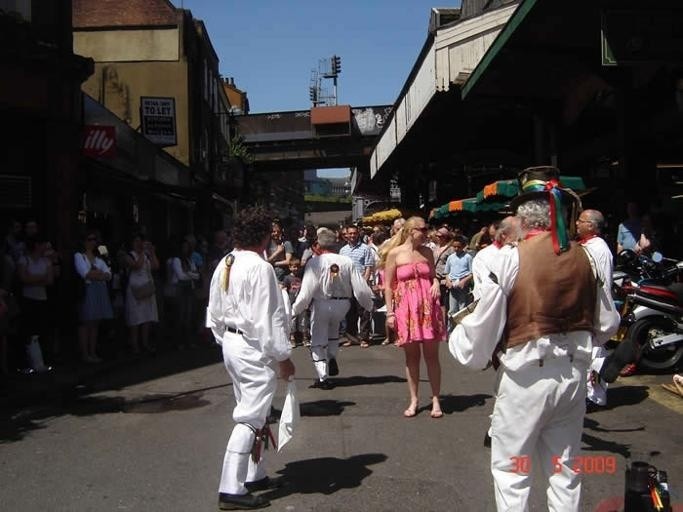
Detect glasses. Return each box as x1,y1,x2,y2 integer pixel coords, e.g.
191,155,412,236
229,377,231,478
417,227,428,233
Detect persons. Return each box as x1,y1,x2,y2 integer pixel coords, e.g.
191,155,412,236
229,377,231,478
206,194,665,512
1,219,232,378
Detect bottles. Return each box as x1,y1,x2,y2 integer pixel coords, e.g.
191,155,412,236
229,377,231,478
368,272,375,286
623,461,654,512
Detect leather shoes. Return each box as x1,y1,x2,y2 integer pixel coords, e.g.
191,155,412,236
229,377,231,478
315,359,339,387
243,475,289,492
218,491,270,509
599,338,635,382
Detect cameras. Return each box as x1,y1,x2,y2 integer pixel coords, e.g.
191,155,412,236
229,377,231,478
144,242,152,249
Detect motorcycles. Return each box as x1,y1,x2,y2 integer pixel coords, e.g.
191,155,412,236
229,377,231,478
620,259,683,374
611,249,670,298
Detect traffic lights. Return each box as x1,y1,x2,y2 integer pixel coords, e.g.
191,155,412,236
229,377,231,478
308,86,317,102
334,55,341,74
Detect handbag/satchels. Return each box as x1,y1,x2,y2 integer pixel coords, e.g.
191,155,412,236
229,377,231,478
130,279,156,301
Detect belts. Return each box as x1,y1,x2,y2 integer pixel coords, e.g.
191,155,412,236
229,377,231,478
330,296,350,299
225,326,242,334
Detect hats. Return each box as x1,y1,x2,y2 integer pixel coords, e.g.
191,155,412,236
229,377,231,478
510,166,579,211
